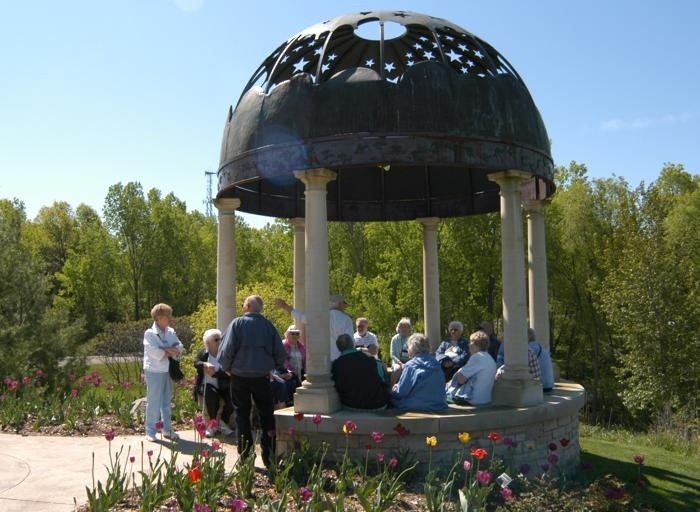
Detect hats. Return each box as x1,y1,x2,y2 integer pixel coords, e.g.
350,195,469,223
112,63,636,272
284,325,302,339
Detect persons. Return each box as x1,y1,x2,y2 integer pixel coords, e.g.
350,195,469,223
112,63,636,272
445,330,496,406
270,325,306,409
141,302,184,442
331,334,389,413
276,299,354,363
353,318,379,361
496,343,541,381
528,328,553,391
390,333,448,413
478,321,501,361
390,316,415,388
216,297,292,468
197,328,234,437
436,321,471,381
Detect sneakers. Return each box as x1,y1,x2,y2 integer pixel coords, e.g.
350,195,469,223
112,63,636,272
204,422,221,437
146,431,156,442
162,430,180,440
218,419,235,436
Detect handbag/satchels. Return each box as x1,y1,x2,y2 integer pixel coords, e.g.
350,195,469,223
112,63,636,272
285,374,302,400
203,383,220,395
167,356,185,382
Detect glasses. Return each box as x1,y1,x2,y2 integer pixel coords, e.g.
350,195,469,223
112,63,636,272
291,333,299,336
